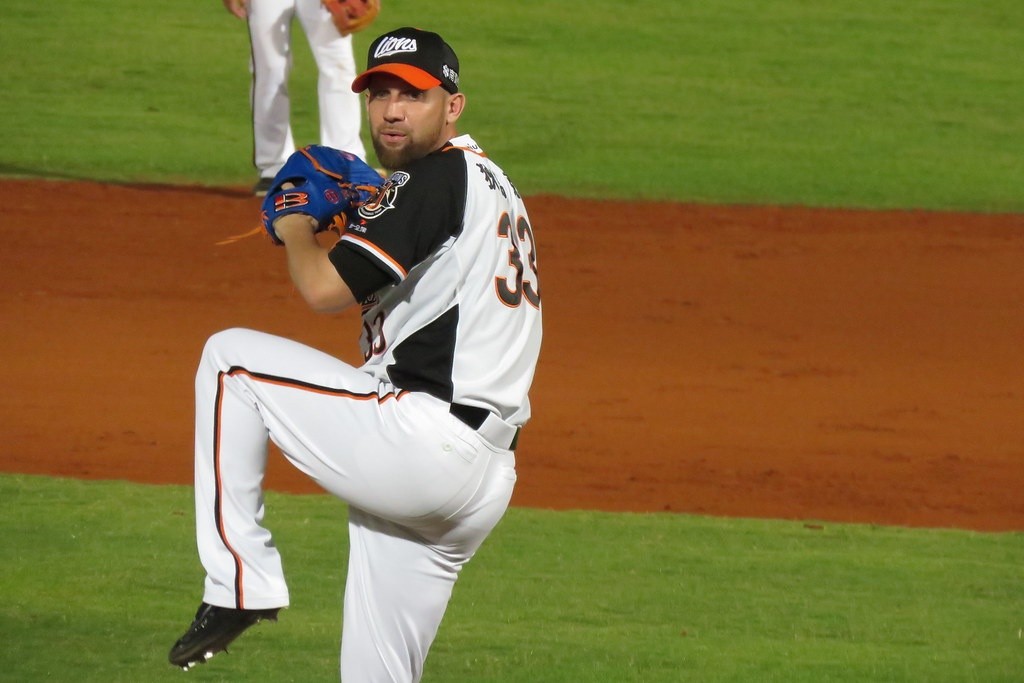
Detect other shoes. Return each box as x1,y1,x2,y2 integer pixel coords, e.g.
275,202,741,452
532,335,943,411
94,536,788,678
254,178,273,197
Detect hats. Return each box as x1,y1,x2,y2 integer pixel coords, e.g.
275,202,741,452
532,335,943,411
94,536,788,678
351,27,459,94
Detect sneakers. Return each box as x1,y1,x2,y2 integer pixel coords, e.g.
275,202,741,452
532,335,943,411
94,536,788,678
169,602,279,667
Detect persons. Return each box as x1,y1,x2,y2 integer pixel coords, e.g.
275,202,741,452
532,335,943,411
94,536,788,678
224,0,380,196
168,27,543,683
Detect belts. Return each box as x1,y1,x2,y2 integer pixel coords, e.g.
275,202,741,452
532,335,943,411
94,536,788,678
450,402,520,450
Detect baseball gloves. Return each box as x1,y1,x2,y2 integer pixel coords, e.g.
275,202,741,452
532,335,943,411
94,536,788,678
319,0,382,39
212,142,387,250
